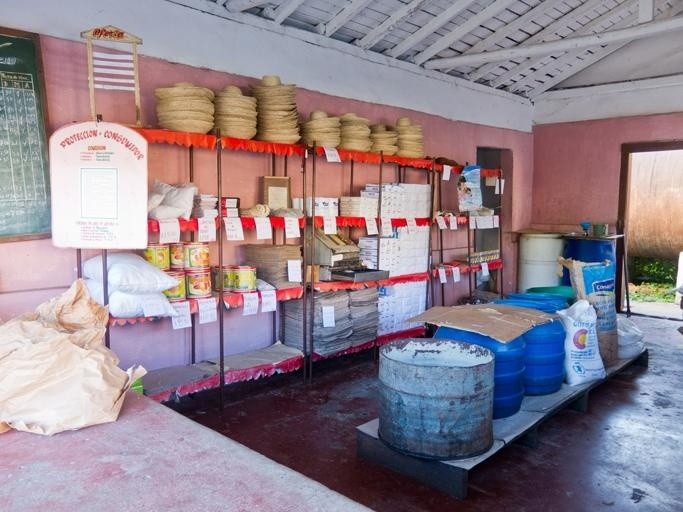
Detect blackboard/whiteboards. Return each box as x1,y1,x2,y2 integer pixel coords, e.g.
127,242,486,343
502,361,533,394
0,29,54,242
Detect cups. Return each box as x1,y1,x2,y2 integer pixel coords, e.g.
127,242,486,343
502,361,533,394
592,224,605,238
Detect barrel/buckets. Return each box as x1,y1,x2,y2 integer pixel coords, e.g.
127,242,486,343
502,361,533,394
491,298,566,396
561,234,618,288
516,229,570,295
430,323,526,420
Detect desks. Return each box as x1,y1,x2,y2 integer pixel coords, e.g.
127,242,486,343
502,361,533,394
1,393,375,512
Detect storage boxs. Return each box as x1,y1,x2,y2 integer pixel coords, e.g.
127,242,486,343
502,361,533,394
291,197,339,218
338,196,378,218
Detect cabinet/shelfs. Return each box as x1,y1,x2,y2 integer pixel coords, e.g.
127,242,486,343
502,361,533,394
431,158,503,334
74,122,309,411
278,138,434,386
